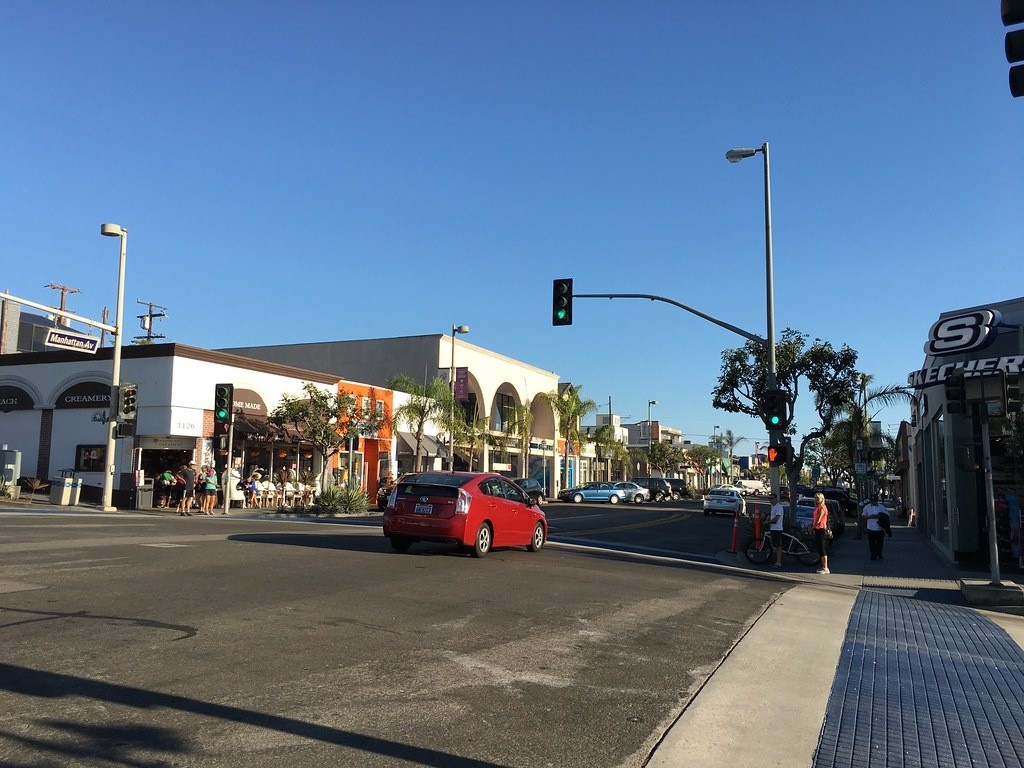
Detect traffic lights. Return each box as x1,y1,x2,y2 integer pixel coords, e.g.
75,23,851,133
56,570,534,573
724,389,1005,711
118,422,133,438
766,388,786,429
944,373,965,416
551,278,573,325
1003,370,1021,414
214,384,232,424
212,435,227,450
768,446,782,467
119,387,138,419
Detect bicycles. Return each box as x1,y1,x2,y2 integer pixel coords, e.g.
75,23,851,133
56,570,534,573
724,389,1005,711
745,515,821,566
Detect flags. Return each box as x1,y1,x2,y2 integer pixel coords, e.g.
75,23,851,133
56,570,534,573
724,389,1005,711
716,442,722,454
454,367,469,404
708,442,715,451
650,421,659,440
435,367,450,401
640,421,649,440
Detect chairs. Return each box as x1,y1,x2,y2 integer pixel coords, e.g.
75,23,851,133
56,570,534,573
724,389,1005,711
244,478,315,508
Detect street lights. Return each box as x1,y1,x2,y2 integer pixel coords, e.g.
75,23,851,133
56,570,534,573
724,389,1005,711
648,400,658,478
727,142,784,531
101,222,128,508
855,439,863,539
714,425,720,450
448,322,469,471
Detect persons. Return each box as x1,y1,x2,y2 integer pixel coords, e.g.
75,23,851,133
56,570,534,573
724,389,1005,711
812,492,832,575
861,494,890,561
145,461,316,513
762,493,785,568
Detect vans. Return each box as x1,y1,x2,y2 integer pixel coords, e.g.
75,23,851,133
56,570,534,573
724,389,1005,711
733,480,764,495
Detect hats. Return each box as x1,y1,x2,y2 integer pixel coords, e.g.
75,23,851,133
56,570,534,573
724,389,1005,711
870,494,879,501
189,460,196,465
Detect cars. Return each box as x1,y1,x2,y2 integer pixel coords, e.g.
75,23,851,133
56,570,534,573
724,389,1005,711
376,478,396,510
383,471,548,558
496,478,543,506
703,488,747,516
557,481,650,504
781,484,858,540
703,484,749,496
763,483,771,495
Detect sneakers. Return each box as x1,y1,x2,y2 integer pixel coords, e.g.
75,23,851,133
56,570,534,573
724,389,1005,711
816,567,830,575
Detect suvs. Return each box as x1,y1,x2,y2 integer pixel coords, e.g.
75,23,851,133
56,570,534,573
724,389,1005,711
661,478,687,501
626,477,669,503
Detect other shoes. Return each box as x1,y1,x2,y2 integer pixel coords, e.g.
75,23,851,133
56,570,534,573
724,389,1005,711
157,504,280,516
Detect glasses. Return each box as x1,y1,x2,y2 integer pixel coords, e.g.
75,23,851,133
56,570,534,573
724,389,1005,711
768,497,775,500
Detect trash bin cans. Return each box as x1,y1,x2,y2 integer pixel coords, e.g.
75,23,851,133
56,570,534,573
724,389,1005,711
49,468,82,506
136,478,154,510
